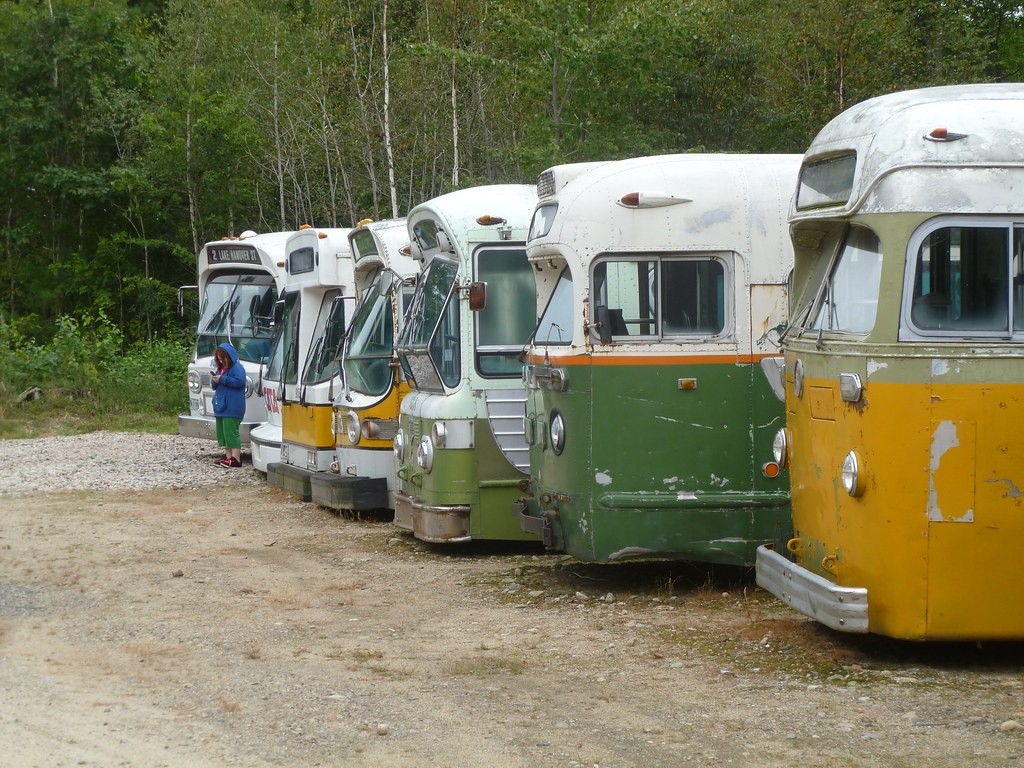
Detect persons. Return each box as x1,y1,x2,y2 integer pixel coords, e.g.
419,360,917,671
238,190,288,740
651,269,697,335
210,342,247,467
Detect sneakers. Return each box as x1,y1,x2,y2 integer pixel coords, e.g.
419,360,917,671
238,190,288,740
221,456,242,468
213,454,228,467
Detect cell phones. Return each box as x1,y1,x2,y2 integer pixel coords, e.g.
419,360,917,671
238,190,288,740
210,371,216,376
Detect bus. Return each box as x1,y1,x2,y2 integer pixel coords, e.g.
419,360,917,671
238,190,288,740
311,217,420,511
267,224,360,502
250,318,282,474
177,230,300,443
754,84,1024,642
391,184,543,543
519,154,797,570
